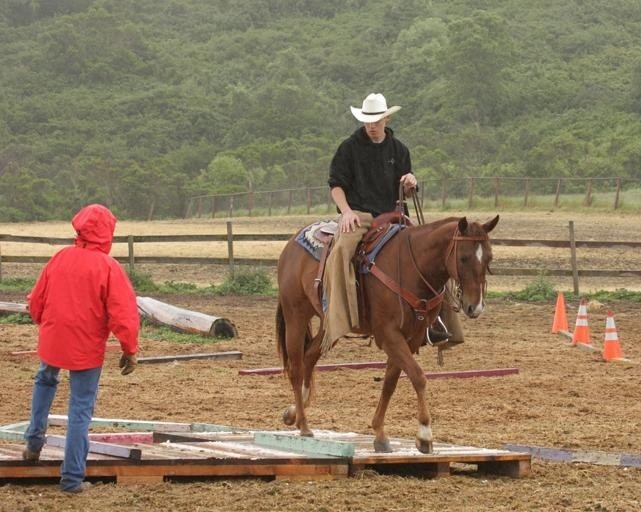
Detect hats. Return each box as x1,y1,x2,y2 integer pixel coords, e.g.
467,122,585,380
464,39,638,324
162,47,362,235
349,93,403,124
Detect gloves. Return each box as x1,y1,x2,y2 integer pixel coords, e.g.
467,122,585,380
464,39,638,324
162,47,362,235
118,354,138,376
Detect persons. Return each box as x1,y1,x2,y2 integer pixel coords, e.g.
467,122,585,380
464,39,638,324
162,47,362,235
20,202,140,496
327,90,455,349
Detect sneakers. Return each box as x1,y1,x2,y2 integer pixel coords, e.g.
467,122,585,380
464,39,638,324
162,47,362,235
427,327,453,343
23,448,41,462
67,481,95,496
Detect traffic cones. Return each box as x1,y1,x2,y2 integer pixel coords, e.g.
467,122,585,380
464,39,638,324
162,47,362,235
549,288,571,336
603,309,626,364
569,296,594,349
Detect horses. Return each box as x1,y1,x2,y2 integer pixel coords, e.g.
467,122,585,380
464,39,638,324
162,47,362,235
275,211,500,454
